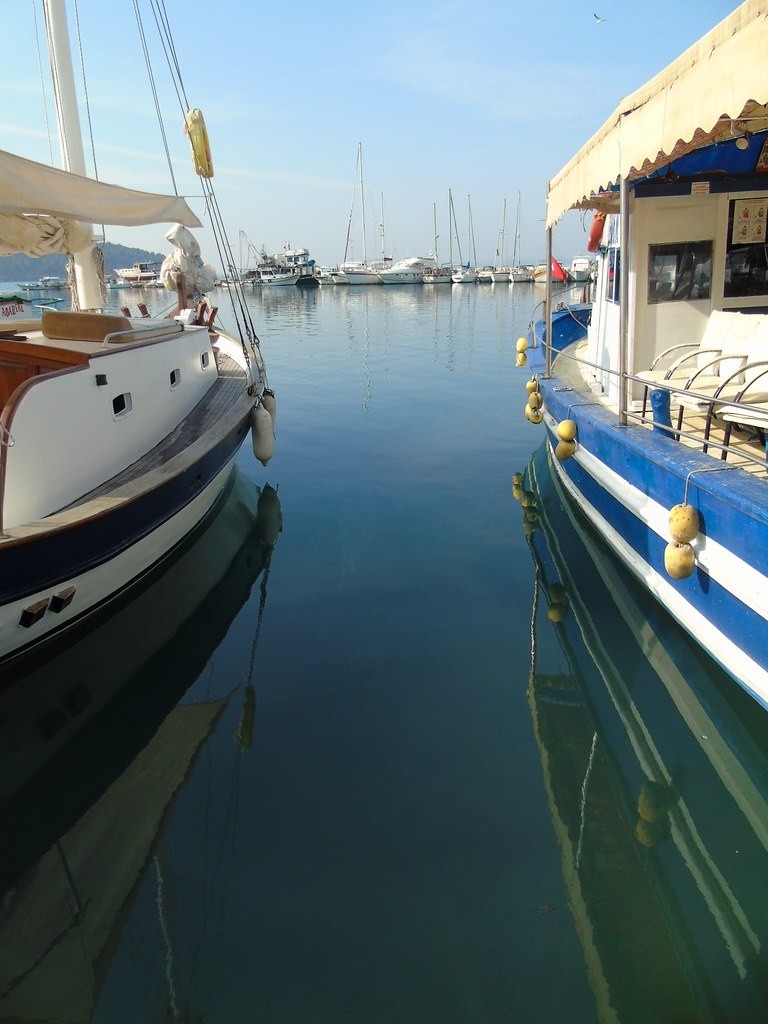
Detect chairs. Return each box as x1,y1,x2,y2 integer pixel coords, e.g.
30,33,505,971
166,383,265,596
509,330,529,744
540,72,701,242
637,310,768,479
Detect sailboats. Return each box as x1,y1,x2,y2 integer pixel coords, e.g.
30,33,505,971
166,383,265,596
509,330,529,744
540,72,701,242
0,0,275,667
213,141,620,286
0,464,284,1024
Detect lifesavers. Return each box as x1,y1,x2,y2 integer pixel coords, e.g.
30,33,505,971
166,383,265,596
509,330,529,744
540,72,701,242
268,279,271,283
260,280,263,283
252,280,255,283
260,265,265,269
587,194,609,253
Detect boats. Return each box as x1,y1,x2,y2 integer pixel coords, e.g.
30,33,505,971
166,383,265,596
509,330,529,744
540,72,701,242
514,435,768,1024
17,276,70,289
104,260,165,289
514,0,768,711
